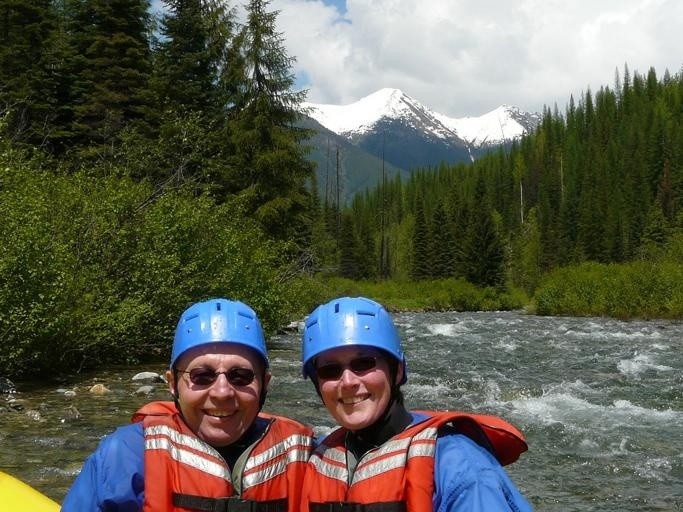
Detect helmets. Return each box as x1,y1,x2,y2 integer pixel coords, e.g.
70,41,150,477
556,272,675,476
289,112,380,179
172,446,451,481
302,296,407,386
170,299,269,374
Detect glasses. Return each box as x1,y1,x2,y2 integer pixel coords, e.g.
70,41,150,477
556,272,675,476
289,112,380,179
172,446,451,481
315,355,384,379
177,368,258,385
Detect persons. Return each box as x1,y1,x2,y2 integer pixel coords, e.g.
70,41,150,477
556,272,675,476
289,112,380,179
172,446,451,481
299,296,533,512
60,298,321,512
